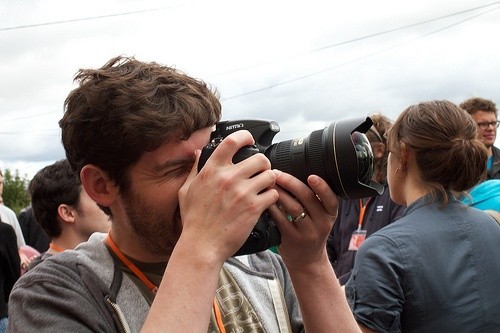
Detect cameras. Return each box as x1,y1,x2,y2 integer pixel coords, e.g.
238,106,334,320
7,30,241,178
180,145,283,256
197,115,386,257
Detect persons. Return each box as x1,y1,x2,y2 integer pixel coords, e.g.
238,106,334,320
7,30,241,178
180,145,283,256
346,101,496,332
328,115,407,280
0,107,114,332
9,60,361,331
461,100,496,182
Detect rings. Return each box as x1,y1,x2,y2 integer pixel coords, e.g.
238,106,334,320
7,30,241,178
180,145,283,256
292,211,307,223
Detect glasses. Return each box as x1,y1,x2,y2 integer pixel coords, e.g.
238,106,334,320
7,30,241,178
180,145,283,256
477,121,500,130
